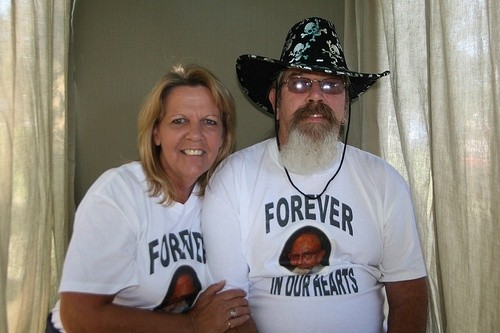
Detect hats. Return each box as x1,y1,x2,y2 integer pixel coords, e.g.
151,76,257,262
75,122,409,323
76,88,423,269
235,17,390,118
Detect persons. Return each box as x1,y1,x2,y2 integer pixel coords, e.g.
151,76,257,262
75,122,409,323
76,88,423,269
45,65,249,333
202,16,430,333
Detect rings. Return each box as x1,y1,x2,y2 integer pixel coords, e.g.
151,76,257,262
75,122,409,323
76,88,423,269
225,322,232,328
228,309,236,317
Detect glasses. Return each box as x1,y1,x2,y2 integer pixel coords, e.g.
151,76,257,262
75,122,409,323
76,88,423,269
280,75,351,94
